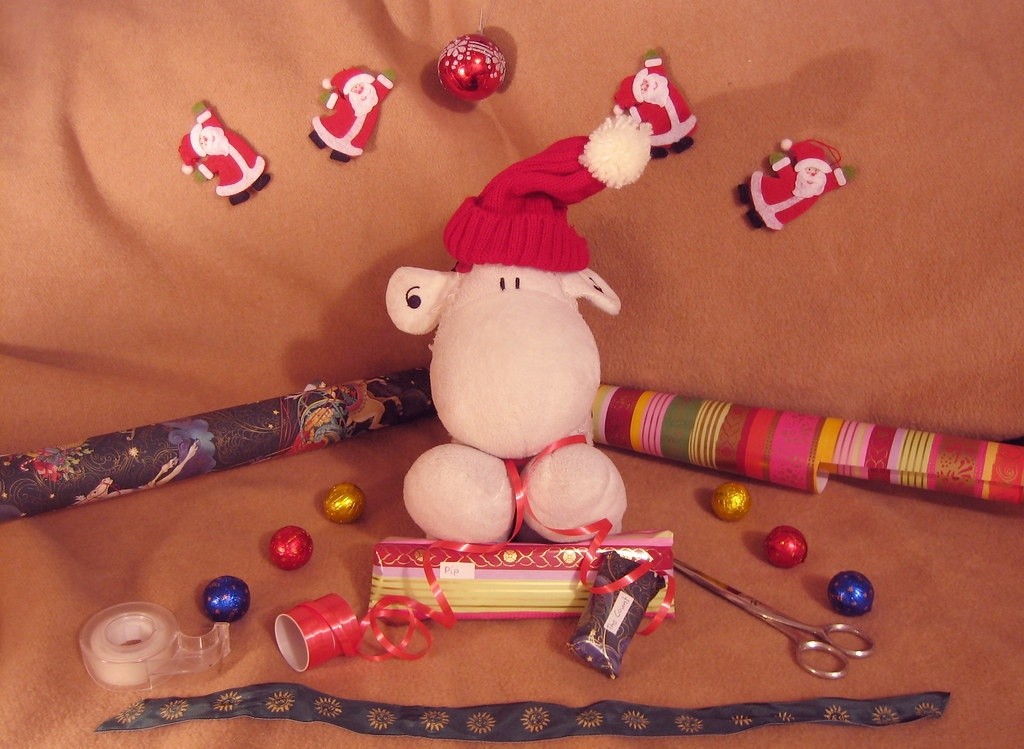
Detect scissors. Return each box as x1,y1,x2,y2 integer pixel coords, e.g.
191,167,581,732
668,556,879,681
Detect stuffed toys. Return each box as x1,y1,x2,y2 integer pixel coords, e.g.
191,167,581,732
385,101,655,541
737,137,847,230
309,64,394,162
177,98,271,206
612,47,700,159
